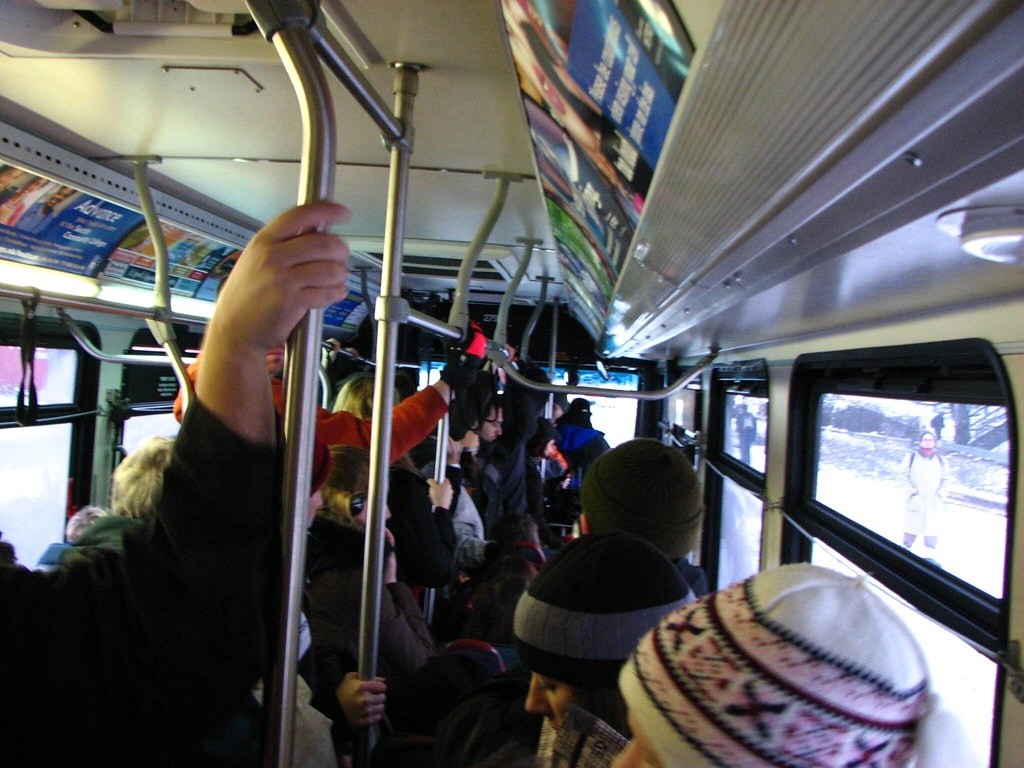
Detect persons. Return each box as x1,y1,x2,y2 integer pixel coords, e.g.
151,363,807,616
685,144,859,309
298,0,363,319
610,563,935,768
932,412,945,440
512,531,697,768
579,438,710,599
63,272,611,768
737,404,756,465
0,200,354,768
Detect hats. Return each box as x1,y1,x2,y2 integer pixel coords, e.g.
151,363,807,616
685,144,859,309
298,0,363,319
581,438,702,555
619,562,929,768
514,529,699,687
310,435,331,494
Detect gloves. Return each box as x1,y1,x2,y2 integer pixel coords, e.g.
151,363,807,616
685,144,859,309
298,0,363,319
441,320,487,393
